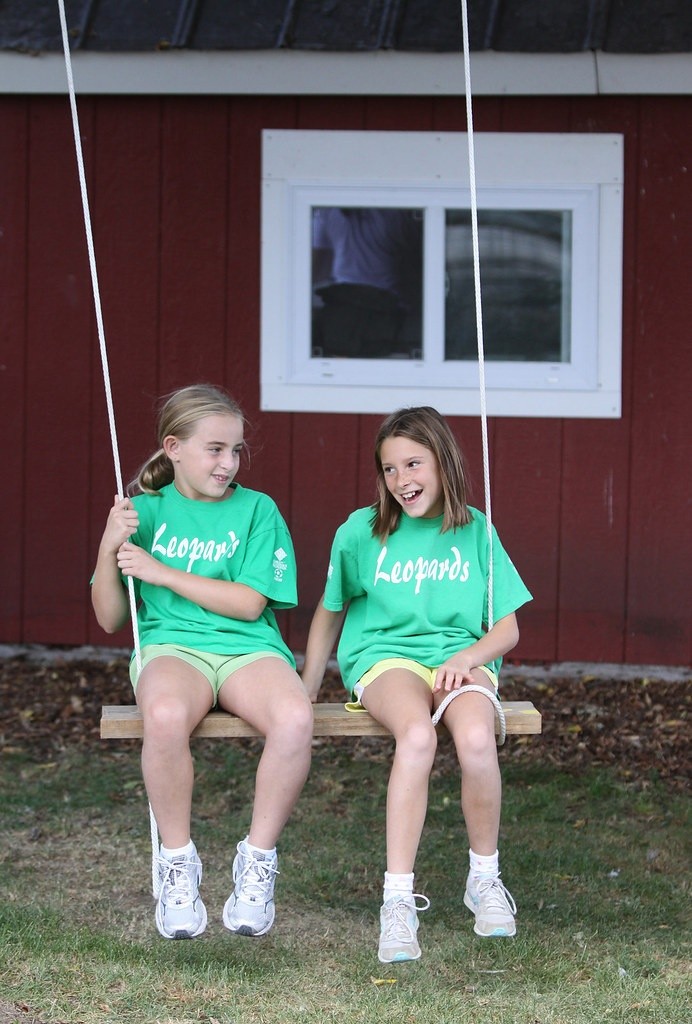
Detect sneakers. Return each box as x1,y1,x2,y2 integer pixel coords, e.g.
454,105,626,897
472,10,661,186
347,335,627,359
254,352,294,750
222,839,281,939
154,841,208,940
462,867,518,938
377,893,431,963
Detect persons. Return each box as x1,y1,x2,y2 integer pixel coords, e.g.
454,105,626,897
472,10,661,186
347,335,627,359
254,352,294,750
89,383,315,940
301,407,534,963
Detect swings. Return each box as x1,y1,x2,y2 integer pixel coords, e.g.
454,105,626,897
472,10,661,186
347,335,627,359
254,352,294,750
56,0,544,738
